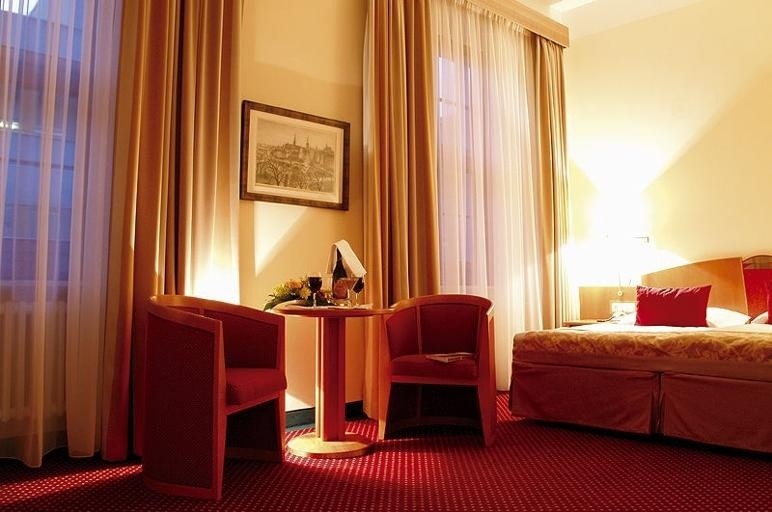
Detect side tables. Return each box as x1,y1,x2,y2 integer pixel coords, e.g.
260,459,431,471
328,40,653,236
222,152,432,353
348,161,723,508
277,298,394,459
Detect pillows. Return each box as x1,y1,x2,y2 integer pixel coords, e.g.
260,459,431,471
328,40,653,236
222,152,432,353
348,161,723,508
634,284,711,327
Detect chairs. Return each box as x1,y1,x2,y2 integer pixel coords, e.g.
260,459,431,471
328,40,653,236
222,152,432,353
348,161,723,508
138,294,287,501
376,294,497,451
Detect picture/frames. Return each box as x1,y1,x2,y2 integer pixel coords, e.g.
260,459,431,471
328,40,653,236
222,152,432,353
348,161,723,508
240,99,350,213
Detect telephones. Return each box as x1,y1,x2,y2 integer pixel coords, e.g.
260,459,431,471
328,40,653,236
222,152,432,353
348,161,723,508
611,312,627,324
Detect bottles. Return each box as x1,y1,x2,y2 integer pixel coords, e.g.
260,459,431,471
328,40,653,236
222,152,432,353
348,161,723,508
332,247,349,305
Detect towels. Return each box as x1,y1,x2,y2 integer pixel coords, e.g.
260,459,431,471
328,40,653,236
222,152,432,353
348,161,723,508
325,238,367,292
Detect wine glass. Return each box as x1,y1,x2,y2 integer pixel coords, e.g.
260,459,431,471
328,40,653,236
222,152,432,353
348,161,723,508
306,270,364,309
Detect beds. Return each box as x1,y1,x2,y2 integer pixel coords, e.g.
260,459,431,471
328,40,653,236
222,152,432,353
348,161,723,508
508,255,772,452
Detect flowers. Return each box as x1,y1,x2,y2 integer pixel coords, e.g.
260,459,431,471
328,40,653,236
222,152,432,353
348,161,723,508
262,273,339,310
